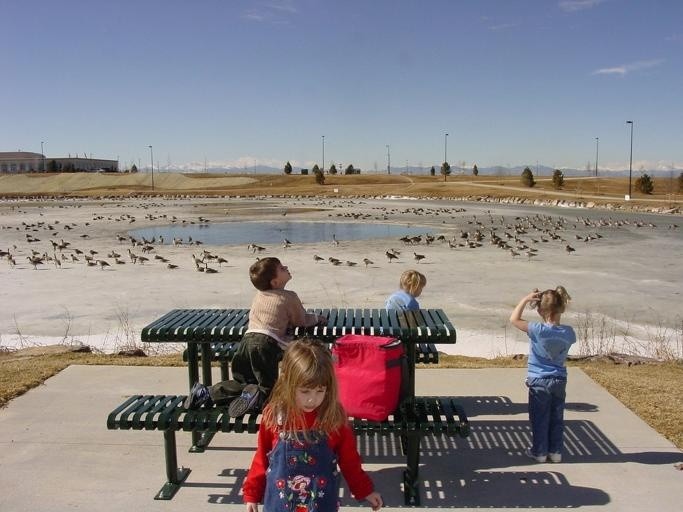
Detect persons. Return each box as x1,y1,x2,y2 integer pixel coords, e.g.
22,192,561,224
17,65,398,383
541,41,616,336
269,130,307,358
509,285,578,466
181,254,329,419
382,268,427,312
239,337,386,512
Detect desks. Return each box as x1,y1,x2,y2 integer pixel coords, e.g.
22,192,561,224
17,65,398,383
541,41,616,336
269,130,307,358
140,309,457,454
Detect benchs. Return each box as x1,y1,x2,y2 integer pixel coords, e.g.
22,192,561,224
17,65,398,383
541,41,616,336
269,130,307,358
106,394,468,506
183,340,439,392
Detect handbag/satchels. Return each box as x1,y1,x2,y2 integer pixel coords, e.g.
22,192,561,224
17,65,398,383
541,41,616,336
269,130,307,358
331,333,408,421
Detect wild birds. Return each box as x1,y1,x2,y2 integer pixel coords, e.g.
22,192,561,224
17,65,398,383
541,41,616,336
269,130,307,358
248,244,256,250
329,257,342,265
363,258,373,267
313,255,324,263
400,206,679,264
0,212,228,274
252,246,265,253
386,248,400,263
282,238,292,248
347,260,357,266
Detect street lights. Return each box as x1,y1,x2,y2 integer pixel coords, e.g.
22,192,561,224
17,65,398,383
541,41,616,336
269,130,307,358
626,120,634,199
147,145,155,191
385,144,390,176
594,137,599,177
444,132,449,182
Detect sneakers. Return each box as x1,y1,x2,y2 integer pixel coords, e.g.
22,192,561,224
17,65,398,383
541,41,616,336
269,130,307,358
525,447,562,462
184,382,210,409
228,383,260,417
